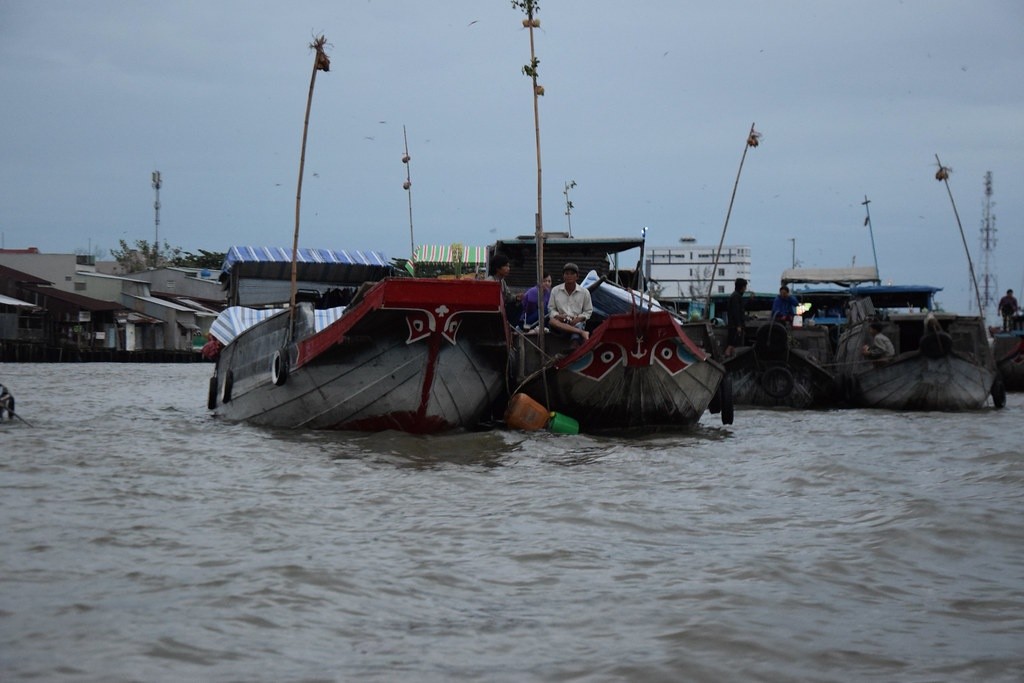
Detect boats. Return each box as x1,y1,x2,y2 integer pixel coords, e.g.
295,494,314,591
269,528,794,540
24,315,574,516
208,32,511,434
491,0,1024,433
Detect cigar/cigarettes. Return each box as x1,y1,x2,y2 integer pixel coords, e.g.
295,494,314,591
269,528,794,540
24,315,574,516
739,333,740,336
563,318,566,322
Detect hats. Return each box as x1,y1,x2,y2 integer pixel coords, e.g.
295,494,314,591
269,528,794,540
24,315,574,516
562,263,579,277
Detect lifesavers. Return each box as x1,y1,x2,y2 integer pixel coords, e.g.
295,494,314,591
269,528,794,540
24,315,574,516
990,379,1006,409
754,321,789,359
761,365,793,399
919,330,954,357
707,373,735,425
221,369,234,404
208,376,218,410
271,347,289,386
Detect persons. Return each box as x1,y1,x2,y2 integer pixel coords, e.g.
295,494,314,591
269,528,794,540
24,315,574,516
862,322,895,360
485,255,594,352
728,278,755,347
771,287,800,323
998,289,1018,332
